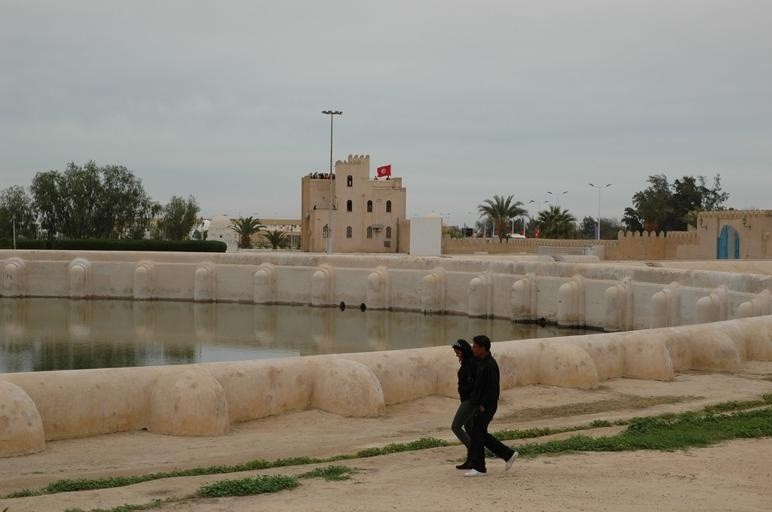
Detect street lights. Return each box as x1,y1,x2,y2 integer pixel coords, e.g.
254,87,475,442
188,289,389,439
322,107,343,255
587,180,613,240
529,180,569,238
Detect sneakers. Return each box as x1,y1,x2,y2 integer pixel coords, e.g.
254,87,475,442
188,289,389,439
505,452,518,471
456,461,487,477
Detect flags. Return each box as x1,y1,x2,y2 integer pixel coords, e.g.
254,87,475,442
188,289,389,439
377,164,391,177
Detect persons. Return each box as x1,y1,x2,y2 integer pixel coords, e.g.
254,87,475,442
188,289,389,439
310,172,330,179
451,335,519,477
